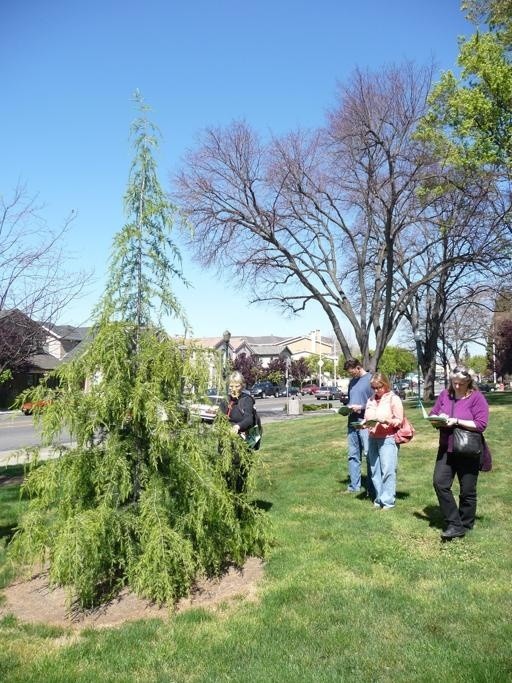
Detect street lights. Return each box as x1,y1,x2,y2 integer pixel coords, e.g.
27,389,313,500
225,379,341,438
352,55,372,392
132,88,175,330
223,329,231,404
480,321,497,383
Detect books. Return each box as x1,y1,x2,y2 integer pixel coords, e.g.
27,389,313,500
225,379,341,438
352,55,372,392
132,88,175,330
349,420,375,428
417,399,447,427
329,406,351,417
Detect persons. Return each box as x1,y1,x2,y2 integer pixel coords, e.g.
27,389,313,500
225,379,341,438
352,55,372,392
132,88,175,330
339,358,374,494
428,367,492,539
361,372,404,511
217,371,262,520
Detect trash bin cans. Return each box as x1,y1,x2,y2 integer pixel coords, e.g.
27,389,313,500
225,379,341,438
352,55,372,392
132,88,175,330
288,395,303,415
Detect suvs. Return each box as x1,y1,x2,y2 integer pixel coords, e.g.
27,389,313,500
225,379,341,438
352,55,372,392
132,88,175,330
193,388,228,396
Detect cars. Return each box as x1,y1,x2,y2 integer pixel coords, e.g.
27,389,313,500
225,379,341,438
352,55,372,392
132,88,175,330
279,387,299,397
20,396,53,415
314,386,345,400
186,395,227,423
476,382,498,391
393,371,446,399
300,383,318,395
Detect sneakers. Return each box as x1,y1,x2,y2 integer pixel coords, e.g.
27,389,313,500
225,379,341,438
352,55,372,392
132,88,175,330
441,527,465,539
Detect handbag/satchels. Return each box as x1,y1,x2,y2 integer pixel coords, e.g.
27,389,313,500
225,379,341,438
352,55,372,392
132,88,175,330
248,413,262,450
453,427,483,457
390,394,416,444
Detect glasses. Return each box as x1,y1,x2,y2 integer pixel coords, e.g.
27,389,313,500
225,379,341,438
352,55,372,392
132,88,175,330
453,369,471,377
371,383,384,389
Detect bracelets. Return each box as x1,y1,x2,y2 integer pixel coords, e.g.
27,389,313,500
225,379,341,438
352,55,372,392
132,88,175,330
456,417,460,426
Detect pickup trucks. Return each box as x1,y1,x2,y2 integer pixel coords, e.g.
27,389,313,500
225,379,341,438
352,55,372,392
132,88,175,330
248,380,280,398
